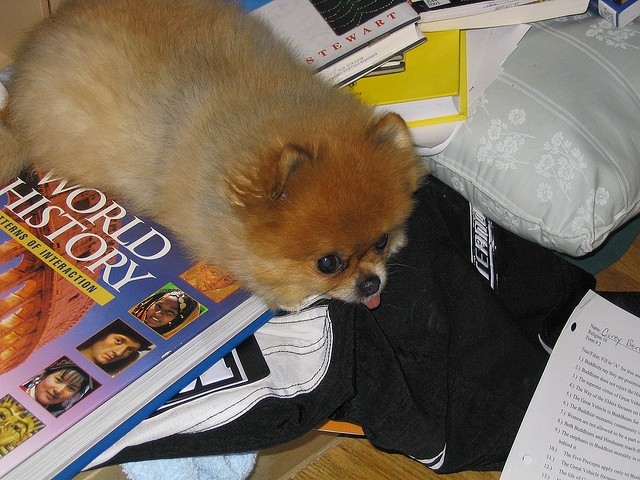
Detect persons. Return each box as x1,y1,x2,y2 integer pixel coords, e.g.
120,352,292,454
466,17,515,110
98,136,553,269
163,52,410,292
20,356,94,418
76,318,153,376
132,289,200,339
0,393,46,460
195,259,237,292
588,0,640,28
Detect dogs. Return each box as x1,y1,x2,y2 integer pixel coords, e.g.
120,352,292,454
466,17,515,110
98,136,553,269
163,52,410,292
8,0,430,312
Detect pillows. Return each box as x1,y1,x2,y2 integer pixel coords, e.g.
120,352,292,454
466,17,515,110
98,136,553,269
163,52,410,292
419,12,640,260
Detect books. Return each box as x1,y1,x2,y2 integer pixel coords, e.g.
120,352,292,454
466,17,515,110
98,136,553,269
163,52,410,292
238,0,428,89
410,0,591,32
341,28,468,128
0,165,277,480
